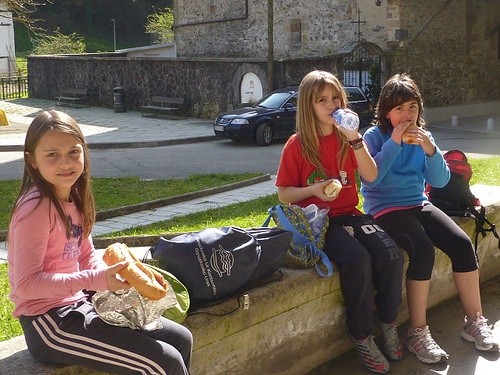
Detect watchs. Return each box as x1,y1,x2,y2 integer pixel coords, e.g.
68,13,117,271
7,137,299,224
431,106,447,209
349,131,364,146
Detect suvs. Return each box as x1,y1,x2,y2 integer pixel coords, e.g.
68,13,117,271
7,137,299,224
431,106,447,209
213,85,374,146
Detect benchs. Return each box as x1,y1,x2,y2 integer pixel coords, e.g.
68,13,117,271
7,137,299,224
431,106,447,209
54,88,90,108
0,183,500,375
140,96,188,120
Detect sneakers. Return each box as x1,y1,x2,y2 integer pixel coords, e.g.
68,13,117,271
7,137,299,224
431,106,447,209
406,324,450,365
460,311,500,352
350,332,390,373
372,311,405,361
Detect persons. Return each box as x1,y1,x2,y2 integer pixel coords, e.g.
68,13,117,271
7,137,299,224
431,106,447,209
275,70,404,373
360,74,500,364
8,109,193,375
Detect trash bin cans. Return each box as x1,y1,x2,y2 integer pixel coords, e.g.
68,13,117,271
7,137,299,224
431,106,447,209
113,87,125,113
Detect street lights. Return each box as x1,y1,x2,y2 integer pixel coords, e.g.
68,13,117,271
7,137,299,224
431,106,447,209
110,18,117,50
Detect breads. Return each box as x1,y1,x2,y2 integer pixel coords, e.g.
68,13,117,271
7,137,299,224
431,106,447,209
402,123,419,144
103,242,168,300
323,179,342,198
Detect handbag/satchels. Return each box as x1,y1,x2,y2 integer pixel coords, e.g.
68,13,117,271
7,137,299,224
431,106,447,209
234,225,293,291
261,202,333,278
153,225,262,306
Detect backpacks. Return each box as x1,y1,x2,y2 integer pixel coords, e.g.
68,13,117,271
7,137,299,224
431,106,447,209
139,260,190,324
424,148,475,218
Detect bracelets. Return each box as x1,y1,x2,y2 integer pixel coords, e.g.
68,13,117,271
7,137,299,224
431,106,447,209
352,142,364,150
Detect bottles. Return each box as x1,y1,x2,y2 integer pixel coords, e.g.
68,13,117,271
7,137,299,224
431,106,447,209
330,110,359,131
